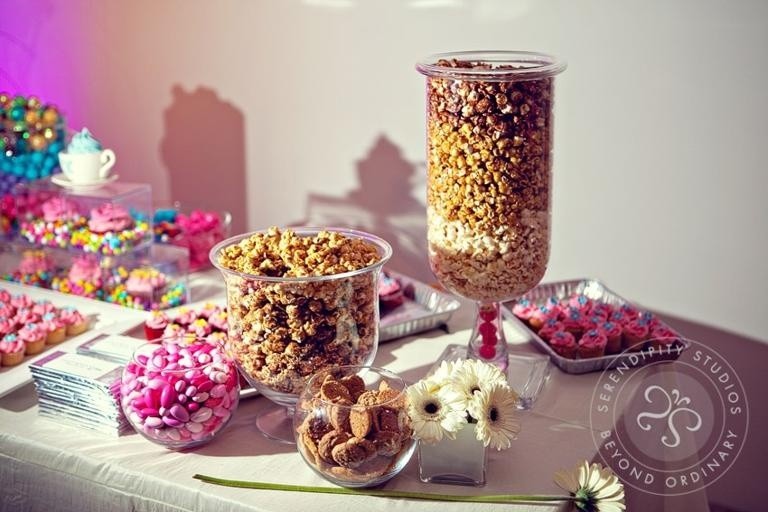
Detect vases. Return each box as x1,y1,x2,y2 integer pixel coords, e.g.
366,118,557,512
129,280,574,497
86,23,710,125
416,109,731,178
417,425,489,484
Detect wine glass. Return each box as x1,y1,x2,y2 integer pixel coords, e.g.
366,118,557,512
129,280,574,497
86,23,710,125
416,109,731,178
206,225,394,447
411,45,571,411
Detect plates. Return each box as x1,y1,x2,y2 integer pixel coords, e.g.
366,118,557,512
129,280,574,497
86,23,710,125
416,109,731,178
51,172,120,192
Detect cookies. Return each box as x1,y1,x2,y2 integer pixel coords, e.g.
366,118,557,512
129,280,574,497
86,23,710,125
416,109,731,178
294,374,412,484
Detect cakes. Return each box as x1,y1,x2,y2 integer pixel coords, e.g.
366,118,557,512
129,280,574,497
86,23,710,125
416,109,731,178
143,302,235,358
0,288,86,366
379,271,406,317
512,293,676,360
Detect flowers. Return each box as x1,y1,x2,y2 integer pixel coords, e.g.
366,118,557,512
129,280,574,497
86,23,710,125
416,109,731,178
403,356,521,450
193,463,627,512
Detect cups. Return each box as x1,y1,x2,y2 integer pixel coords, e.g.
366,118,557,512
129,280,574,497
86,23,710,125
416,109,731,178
118,334,241,451
57,149,117,182
292,364,418,489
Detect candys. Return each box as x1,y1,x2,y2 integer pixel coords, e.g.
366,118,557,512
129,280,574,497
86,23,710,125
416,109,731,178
120,343,241,444
0,93,226,313
